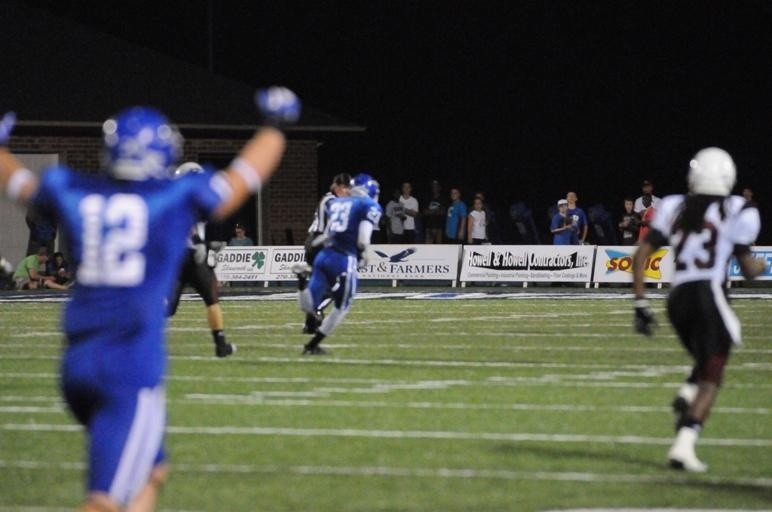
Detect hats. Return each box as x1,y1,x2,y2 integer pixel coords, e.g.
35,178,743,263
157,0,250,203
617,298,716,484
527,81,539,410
558,198,568,204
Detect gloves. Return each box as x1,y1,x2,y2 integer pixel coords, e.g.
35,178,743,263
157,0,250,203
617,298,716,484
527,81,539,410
634,299,657,335
254,84,301,121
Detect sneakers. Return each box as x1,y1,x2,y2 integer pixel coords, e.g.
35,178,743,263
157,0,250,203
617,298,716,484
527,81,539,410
215,342,236,358
302,343,326,356
302,325,319,334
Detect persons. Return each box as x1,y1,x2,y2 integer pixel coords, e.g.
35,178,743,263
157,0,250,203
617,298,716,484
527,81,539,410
0,85,304,512
632,146,767,471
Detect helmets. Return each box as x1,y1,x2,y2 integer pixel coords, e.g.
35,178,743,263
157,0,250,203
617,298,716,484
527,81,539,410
350,173,380,198
98,105,181,165
689,146,738,198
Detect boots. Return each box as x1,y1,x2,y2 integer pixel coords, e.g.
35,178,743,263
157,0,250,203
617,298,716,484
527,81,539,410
674,381,699,413
666,424,708,471
